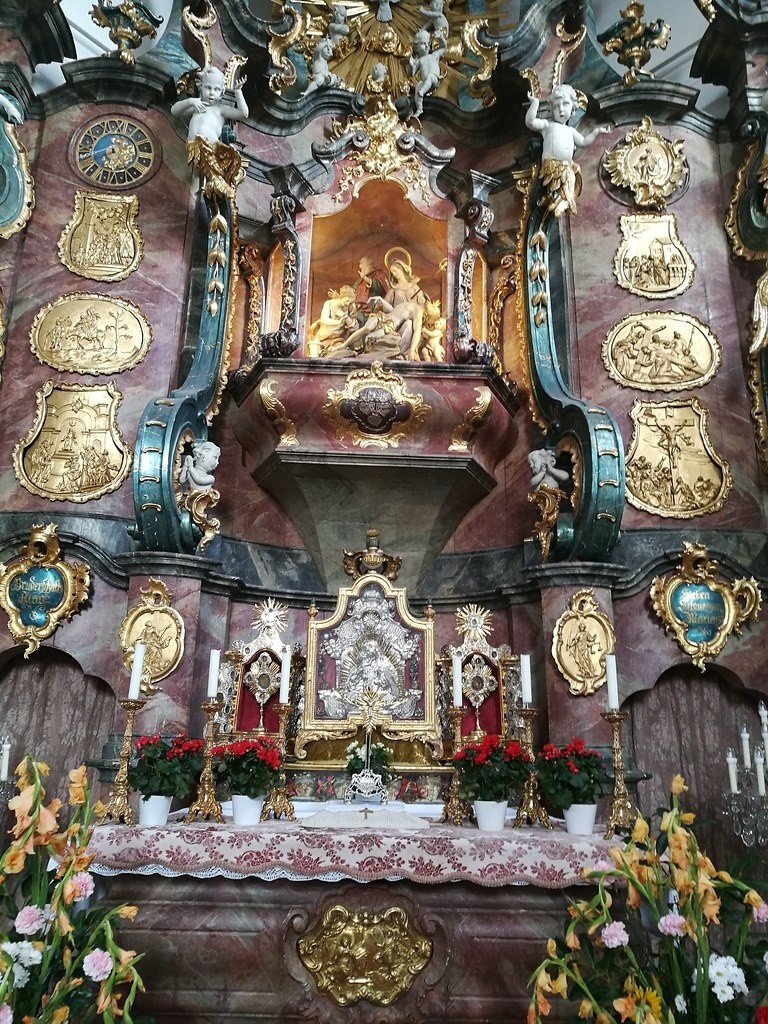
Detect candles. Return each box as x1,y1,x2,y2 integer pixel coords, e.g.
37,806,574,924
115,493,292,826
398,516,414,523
725,700,768,797
206,649,222,698
520,654,532,704
127,642,146,701
0,736,11,782
453,655,462,708
605,651,620,712
278,649,291,704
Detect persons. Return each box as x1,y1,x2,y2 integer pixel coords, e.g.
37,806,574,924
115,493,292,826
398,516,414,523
301,4,349,97
179,441,221,492
171,67,249,139
307,255,447,362
406,0,449,117
524,83,611,161
527,449,569,490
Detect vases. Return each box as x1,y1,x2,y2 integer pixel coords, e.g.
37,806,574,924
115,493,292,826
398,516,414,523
138,793,174,828
472,800,508,831
231,793,263,827
561,803,598,836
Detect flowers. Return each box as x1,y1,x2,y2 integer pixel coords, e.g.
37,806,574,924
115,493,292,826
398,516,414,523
0,752,148,1024
346,738,397,794
209,735,286,800
125,728,202,799
533,737,610,810
453,733,538,803
526,768,767,1024
